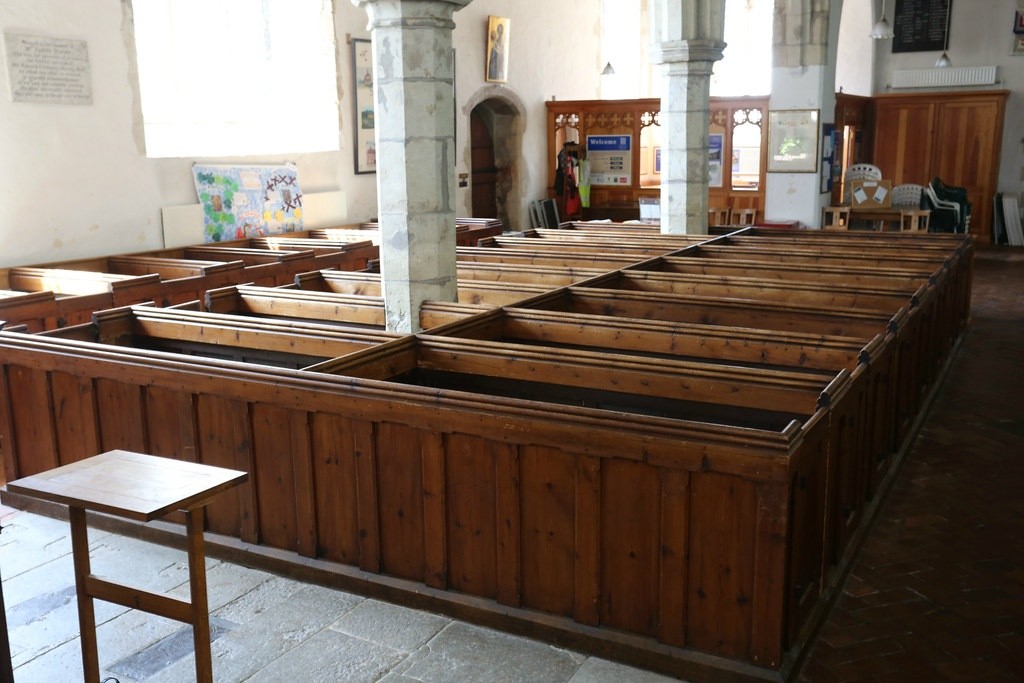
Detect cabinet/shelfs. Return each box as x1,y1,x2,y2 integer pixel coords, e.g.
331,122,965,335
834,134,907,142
872,88,1011,245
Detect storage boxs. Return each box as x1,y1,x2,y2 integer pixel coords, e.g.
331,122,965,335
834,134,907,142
851,178,892,208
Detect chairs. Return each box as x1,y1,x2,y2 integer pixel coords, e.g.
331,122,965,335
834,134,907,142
708,206,758,227
822,207,850,232
843,163,881,202
874,176,971,235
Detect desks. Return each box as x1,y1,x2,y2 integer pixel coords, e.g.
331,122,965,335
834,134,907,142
582,198,639,220
825,203,920,230
5,448,249,683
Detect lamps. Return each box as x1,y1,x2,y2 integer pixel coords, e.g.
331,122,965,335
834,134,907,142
602,60,616,76
934,0,953,67
869,0,896,41
